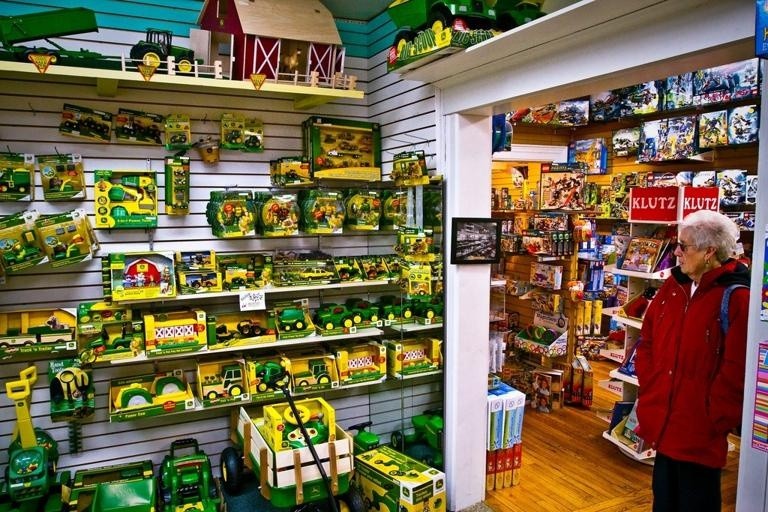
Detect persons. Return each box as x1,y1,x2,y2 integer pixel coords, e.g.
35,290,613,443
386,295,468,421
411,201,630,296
632,208,752,511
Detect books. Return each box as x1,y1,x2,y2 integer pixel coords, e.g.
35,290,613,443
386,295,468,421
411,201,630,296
501,355,592,413
620,227,676,273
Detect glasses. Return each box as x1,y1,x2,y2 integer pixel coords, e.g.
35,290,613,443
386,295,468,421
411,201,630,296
678,242,697,253
